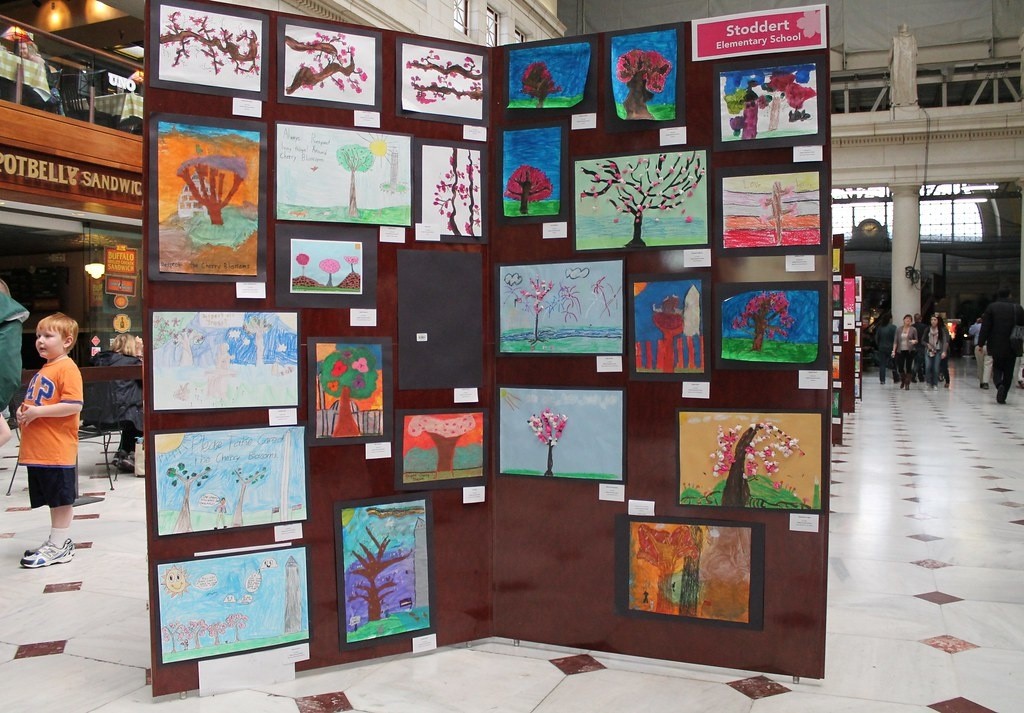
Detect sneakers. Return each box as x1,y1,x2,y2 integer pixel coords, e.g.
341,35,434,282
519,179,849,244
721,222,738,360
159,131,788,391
111,449,135,473
20,538,75,570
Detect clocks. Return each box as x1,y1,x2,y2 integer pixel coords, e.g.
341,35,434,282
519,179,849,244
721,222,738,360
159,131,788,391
856,219,883,243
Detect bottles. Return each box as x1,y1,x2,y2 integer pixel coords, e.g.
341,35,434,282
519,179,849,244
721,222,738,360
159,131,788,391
136,437,144,450
120,317,124,328
929,348,934,357
891,351,895,359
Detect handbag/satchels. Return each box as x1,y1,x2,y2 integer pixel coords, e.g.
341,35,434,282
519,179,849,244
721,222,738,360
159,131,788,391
1009,303,1024,358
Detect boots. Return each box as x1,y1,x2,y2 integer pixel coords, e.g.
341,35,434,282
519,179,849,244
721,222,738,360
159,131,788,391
900,373,912,390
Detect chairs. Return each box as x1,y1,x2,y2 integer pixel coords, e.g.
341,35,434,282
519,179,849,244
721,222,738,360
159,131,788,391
60,74,111,127
80,381,123,490
5,385,79,498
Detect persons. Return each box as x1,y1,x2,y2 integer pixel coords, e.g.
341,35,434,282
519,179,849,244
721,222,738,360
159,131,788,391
84,334,143,473
0,280,29,449
1014,353,1024,389
875,314,900,384
979,286,1024,405
969,313,994,389
911,313,928,383
19,41,59,111
922,314,950,390
892,315,919,390
16,312,83,568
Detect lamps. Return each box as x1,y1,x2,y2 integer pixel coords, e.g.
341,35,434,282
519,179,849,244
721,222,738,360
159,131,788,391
85,243,105,279
1,26,30,57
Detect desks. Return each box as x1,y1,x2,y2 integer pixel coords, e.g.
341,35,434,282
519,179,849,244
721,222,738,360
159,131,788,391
61,92,143,133
0,48,51,105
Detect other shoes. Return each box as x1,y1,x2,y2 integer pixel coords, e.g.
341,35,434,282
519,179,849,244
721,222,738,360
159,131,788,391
880,378,885,384
944,383,949,388
996,384,1005,404
934,385,937,391
979,382,989,391
